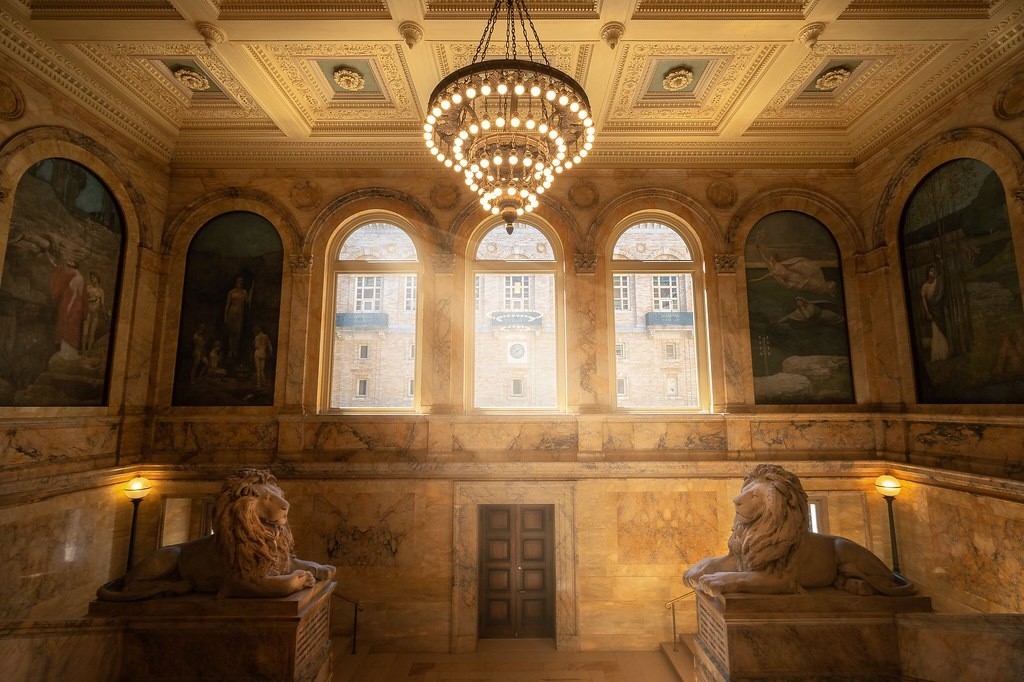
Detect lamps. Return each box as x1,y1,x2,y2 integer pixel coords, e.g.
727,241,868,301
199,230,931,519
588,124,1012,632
873,474,902,575
123,474,152,578
423,0,596,237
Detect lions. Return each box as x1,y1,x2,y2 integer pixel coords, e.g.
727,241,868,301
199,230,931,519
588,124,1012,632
682,464,914,597
96,468,337,602
